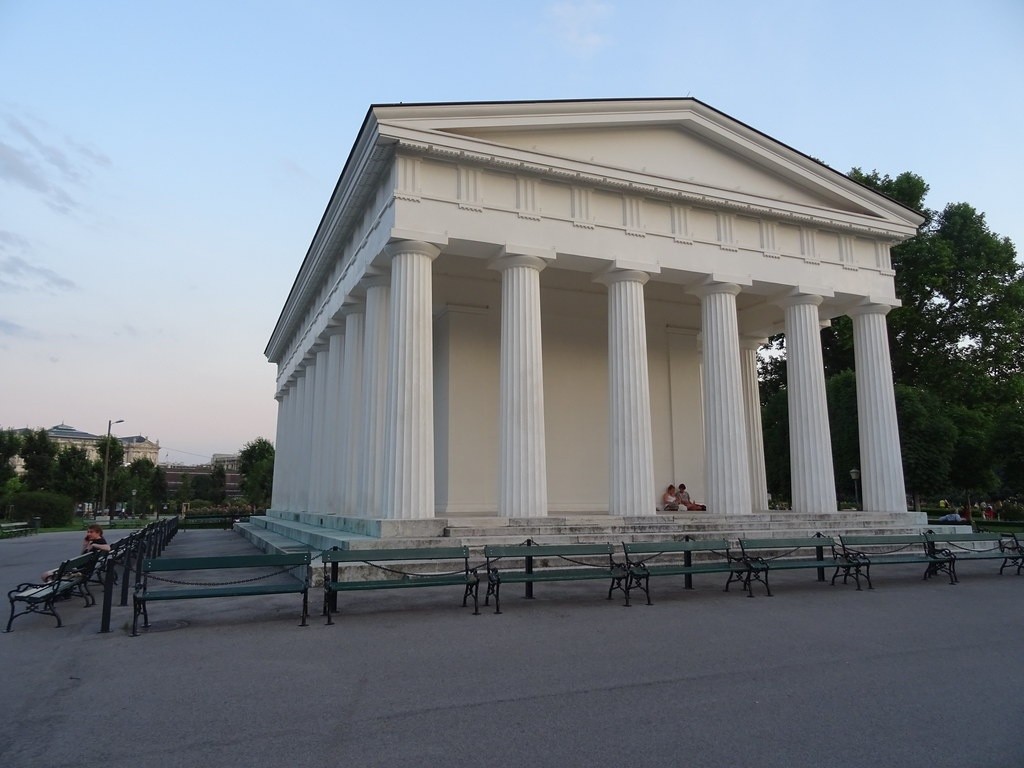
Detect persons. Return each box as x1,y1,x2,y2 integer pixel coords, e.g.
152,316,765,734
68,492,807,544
936,496,993,523
41,524,111,602
664,482,702,511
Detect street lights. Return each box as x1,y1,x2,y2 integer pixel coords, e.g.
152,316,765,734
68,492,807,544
101,419,125,514
849,465,861,505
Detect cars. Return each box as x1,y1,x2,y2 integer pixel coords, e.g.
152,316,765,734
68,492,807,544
104,501,128,517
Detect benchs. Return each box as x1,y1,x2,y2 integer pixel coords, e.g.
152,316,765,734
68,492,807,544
0,516,180,634
483,542,629,616
622,530,1024,610
321,545,482,630
129,550,314,637
